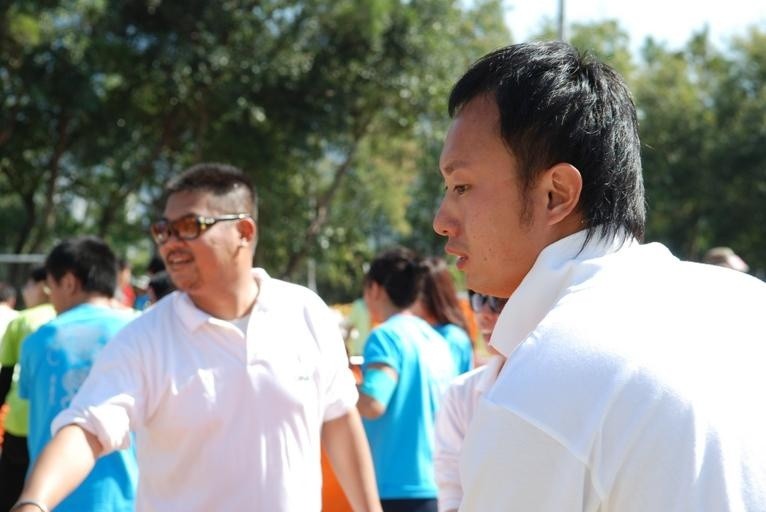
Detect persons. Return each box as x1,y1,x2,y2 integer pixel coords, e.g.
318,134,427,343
433,41,765,512
700,246,749,272
1,162,508,510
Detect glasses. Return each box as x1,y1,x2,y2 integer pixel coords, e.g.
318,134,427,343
150,215,251,245
